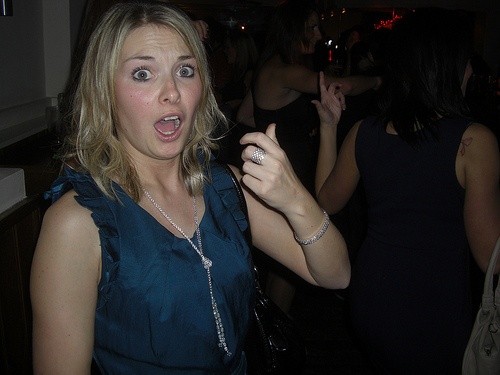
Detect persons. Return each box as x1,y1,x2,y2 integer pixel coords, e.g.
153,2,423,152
203,1,386,197
311,31,499,374
29,2,351,374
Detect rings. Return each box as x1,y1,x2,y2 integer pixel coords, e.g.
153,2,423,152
250,147,267,165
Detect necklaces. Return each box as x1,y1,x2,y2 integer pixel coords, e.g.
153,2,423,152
134,181,233,359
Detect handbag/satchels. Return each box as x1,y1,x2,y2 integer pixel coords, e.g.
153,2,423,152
461,237,500,375
245,266,300,375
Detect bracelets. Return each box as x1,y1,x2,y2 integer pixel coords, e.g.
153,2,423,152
293,209,331,246
372,77,381,92
